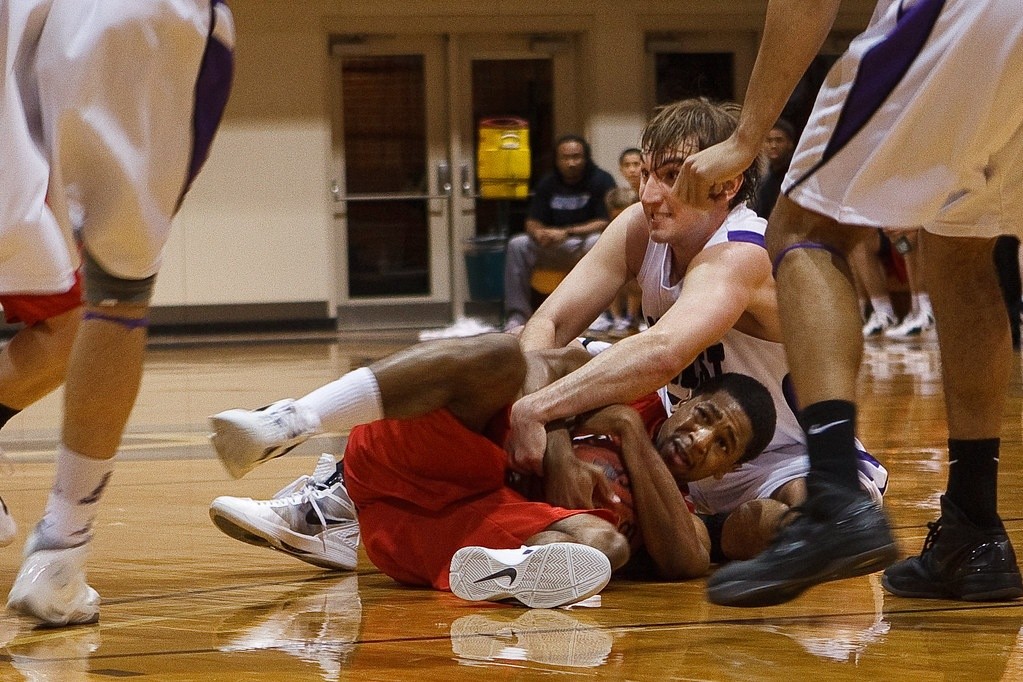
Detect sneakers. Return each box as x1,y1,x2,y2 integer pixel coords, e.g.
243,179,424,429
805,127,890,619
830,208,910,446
452,607,614,668
212,567,364,675
883,295,938,342
0,498,16,547
207,451,361,571
705,493,899,608
862,309,899,337
880,493,1023,601
448,543,612,611
206,398,311,480
4,536,103,626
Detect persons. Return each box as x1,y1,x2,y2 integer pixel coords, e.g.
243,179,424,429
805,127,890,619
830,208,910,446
620,148,642,187
764,119,793,164
503,136,618,326
666,0,1023,607
0,1,236,624
208,334,777,608
210,100,890,571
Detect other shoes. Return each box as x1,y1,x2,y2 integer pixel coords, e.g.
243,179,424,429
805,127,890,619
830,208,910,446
608,319,637,339
